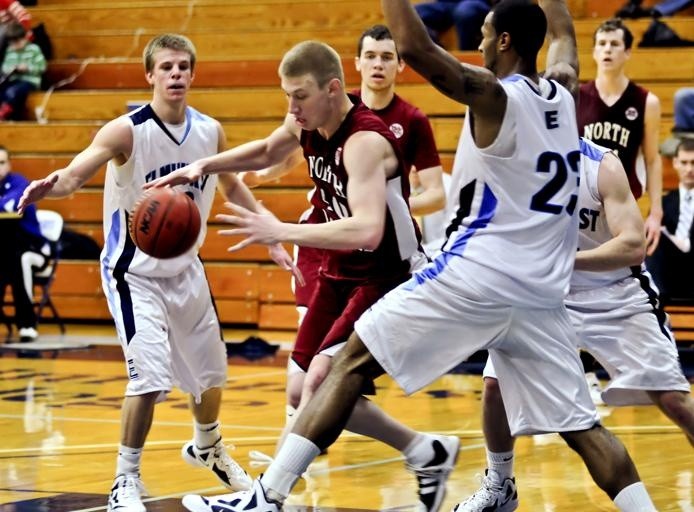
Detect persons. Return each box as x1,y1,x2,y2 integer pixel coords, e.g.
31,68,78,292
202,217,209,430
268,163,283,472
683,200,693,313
18,30,304,511
0,0,63,342
148,0,694,512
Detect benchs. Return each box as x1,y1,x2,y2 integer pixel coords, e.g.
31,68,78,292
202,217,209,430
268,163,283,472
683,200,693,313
0,1,694,341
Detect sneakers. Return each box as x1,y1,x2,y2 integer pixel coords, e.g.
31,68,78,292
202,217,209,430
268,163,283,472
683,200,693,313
246,450,273,467
20,328,37,343
182,435,254,492
451,468,518,512
107,466,148,512
403,435,460,512
181,473,286,512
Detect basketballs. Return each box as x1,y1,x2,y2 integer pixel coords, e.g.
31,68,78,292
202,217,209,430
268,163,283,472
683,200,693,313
128,186,201,258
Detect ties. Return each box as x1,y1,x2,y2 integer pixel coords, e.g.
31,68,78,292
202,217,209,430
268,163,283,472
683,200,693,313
674,194,694,240
